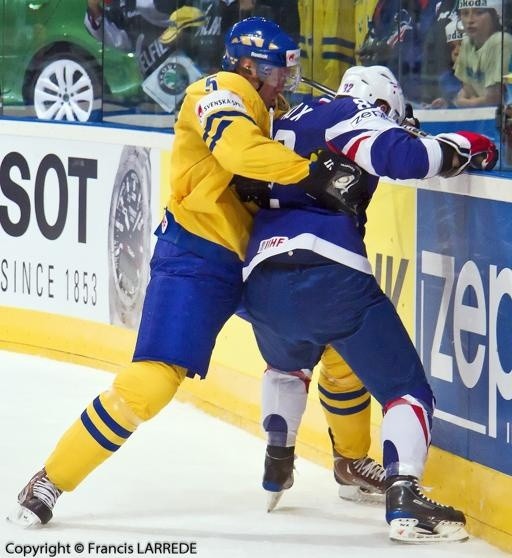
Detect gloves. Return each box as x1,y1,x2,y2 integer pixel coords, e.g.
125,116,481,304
230,172,273,211
402,103,422,140
299,150,372,213
433,130,500,179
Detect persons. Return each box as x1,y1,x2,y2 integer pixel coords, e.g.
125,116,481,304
18,16,386,526
238,64,498,531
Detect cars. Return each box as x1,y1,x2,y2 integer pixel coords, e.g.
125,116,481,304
0,0,156,127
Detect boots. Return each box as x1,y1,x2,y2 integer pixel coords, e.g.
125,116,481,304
384,474,466,534
262,445,295,492
17,467,64,525
331,450,388,495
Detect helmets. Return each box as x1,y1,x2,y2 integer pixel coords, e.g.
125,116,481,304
333,63,407,131
458,0,505,27
219,15,301,92
